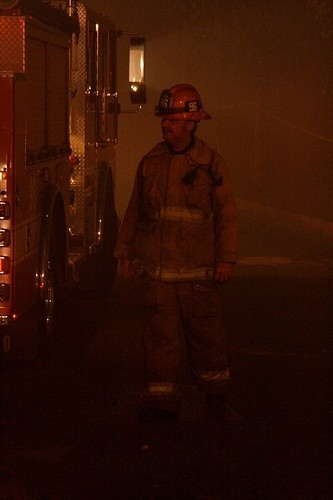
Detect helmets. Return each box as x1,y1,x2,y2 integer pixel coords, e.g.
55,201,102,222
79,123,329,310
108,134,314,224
155,83,211,120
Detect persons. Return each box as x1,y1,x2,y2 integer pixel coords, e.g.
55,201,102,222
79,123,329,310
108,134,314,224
112,84,238,425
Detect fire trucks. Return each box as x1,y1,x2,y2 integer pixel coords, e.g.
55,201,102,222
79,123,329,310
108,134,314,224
0,0,152,363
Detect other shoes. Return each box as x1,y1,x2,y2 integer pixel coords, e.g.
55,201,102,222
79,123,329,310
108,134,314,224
207,394,224,421
139,407,179,421
